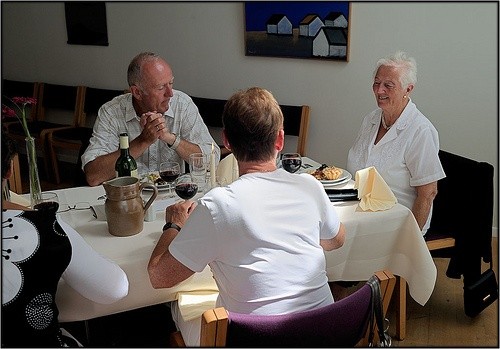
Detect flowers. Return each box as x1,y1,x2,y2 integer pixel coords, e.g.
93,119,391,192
2,97,39,200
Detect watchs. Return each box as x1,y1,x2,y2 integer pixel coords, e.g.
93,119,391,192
163,222,181,231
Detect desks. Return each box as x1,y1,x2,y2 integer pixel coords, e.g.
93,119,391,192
23,157,438,348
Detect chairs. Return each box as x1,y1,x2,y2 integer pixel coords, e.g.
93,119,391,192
1,79,494,348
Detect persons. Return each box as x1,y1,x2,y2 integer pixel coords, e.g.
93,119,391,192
82,53,220,185
148,86,345,349
1,111,129,348
332,53,446,298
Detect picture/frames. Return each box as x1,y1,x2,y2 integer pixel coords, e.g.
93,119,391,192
243,1,351,63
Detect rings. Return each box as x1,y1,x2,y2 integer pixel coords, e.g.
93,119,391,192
157,125,160,130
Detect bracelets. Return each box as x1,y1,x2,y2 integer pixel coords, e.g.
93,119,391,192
167,132,180,149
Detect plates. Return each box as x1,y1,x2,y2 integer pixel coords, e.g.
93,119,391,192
138,173,174,191
298,167,352,187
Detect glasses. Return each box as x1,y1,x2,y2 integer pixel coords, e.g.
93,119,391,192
57,202,97,219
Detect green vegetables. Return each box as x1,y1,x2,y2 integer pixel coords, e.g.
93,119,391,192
316,164,329,171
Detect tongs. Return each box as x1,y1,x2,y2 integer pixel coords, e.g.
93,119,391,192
325,189,358,202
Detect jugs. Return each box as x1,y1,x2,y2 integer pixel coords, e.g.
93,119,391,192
102,176,158,237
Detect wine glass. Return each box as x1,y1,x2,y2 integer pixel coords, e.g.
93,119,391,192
158,161,180,200
175,177,198,203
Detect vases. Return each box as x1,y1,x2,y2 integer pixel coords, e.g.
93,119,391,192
24,137,42,211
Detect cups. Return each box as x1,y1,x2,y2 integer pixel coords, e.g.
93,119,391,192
189,153,228,194
30,192,60,214
282,152,302,174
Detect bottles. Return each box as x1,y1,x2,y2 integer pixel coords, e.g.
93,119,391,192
115,133,138,177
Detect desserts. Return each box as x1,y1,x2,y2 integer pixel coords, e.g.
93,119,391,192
323,165,342,180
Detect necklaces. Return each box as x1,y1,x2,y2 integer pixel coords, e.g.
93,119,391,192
382,113,392,130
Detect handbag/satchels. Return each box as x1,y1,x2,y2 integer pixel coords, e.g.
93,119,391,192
465,270,498,317
